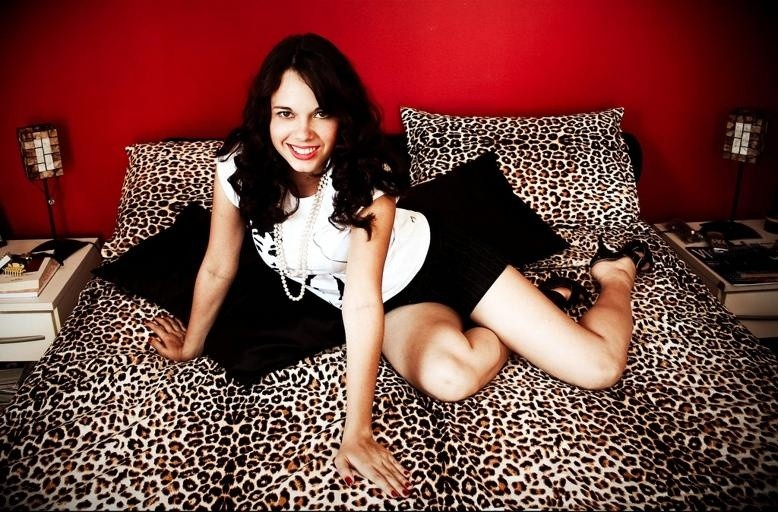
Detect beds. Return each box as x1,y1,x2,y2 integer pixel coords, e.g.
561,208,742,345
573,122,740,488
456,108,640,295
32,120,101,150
0,136,778,511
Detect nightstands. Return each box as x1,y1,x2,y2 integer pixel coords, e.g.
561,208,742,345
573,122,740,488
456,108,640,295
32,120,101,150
2,237,95,363
653,219,777,342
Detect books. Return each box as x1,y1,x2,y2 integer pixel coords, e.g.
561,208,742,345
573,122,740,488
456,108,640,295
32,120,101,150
0,249,58,299
715,248,778,282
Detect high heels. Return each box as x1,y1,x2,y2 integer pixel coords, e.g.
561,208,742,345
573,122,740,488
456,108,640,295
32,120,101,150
537,277,589,309
590,234,654,273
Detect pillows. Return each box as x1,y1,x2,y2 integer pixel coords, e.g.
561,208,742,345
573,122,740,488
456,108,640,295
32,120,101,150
99,199,265,328
100,136,236,267
395,151,571,268
399,101,645,235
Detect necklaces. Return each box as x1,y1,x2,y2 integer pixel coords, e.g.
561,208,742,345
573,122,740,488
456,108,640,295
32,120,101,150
273,157,331,301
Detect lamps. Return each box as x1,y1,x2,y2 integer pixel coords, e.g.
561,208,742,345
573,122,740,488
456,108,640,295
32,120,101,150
700,110,765,239
15,125,89,266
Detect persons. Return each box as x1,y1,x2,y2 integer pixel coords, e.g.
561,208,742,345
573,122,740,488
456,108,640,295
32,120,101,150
144,31,654,499
